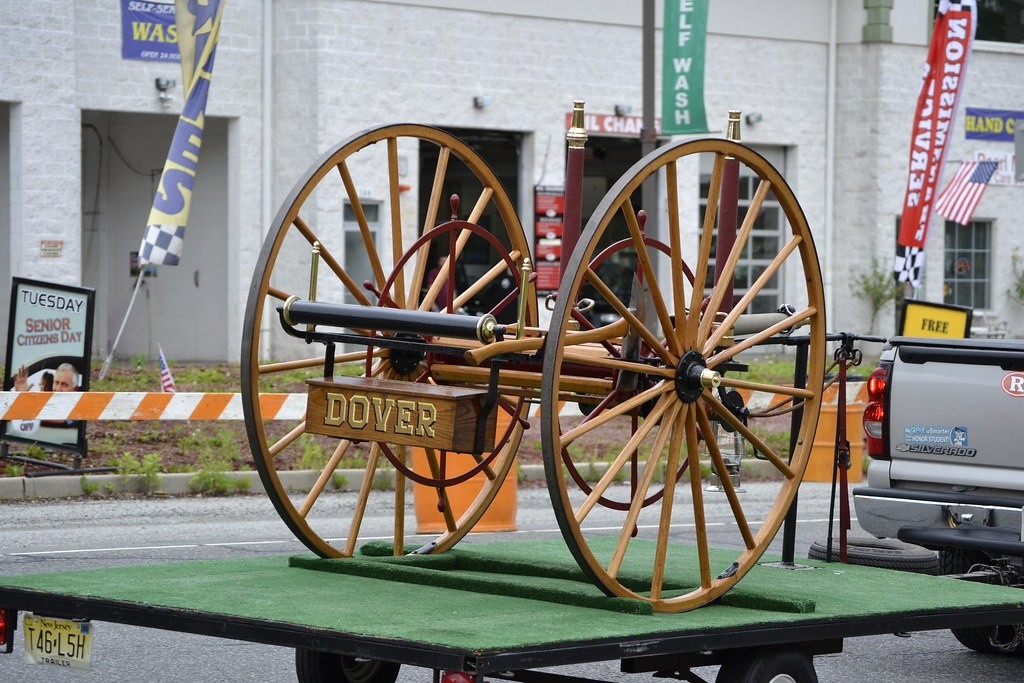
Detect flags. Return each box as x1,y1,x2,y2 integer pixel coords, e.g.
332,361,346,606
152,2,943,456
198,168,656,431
158,350,175,392
936,161,998,226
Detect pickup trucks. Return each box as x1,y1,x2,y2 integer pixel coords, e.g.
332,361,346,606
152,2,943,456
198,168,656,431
852,334,1024,656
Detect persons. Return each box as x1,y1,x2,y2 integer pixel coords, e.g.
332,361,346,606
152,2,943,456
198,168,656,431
39,372,53,391
14,363,78,428
